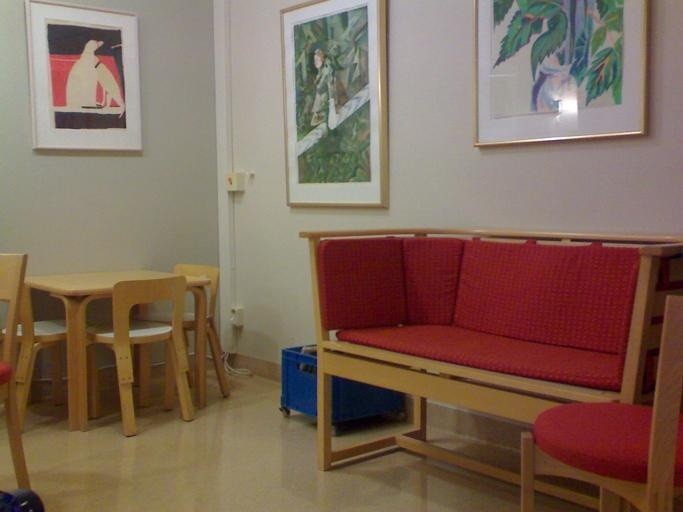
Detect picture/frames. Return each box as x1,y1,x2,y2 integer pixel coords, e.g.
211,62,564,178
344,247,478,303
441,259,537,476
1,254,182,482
470,0,649,149
26,1,145,153
279,0,390,208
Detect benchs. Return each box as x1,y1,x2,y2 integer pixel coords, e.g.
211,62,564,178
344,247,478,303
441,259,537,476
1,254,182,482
296,221,682,510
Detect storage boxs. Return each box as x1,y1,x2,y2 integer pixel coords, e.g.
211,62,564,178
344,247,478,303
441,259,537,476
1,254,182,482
276,330,406,438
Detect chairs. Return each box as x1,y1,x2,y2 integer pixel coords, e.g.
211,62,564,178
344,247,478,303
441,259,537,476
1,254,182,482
133,264,232,409
1,248,41,496
84,274,205,436
517,291,681,512
1,281,102,429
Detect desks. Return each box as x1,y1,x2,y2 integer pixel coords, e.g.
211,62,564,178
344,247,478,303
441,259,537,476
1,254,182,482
23,270,214,431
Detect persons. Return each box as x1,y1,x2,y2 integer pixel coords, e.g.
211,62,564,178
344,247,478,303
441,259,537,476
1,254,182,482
307,48,336,132
